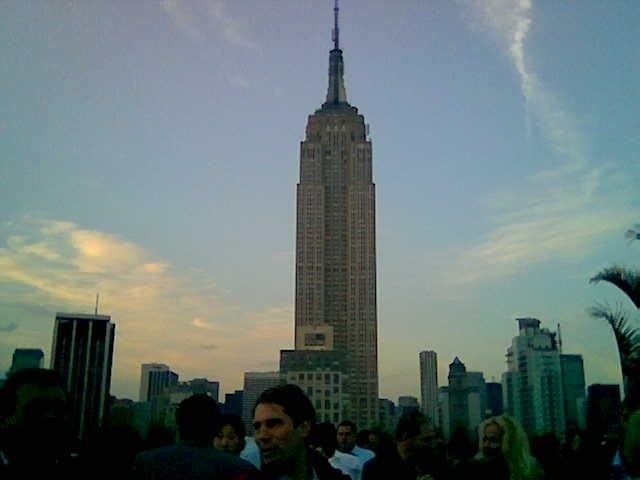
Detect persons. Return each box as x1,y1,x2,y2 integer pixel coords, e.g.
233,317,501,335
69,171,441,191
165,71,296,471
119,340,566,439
113,383,639,479
1,366,83,479
